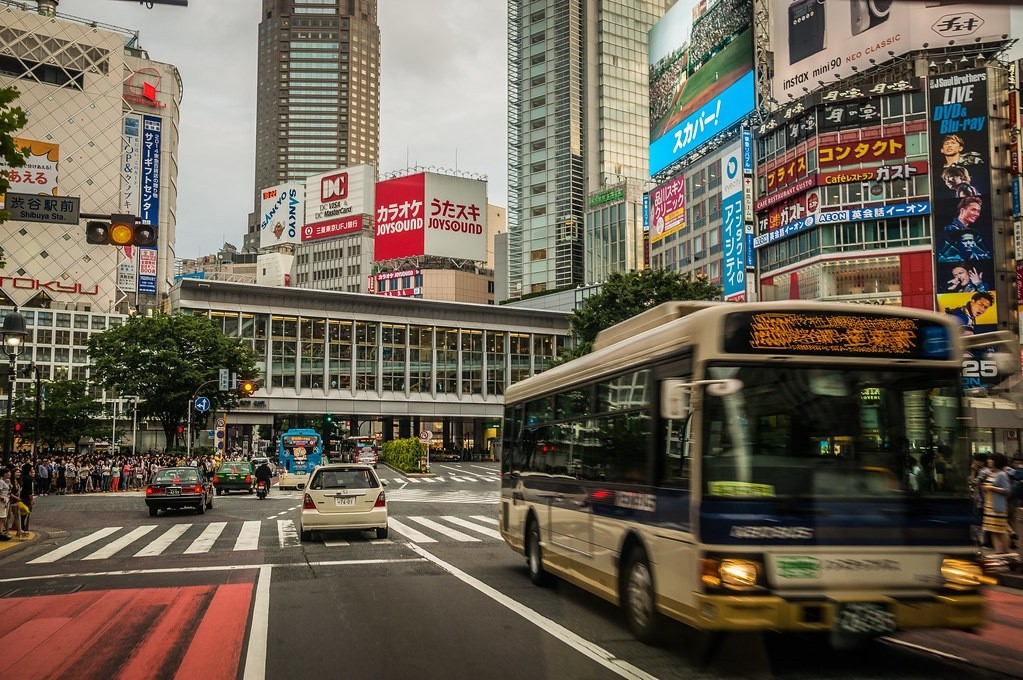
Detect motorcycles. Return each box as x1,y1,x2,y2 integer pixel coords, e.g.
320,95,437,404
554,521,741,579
256,482,270,499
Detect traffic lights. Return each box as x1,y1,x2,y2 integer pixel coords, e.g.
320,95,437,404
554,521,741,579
346,421,350,426
243,382,253,392
11,420,21,434
327,416,331,422
177,426,183,433
195,422,201,439
86,221,157,249
138,422,148,432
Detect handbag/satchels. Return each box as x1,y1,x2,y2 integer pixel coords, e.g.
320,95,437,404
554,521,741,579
17,501,30,515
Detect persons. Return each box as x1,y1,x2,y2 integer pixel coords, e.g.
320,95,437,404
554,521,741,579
0,445,258,545
939,263,988,293
255,460,273,494
941,198,984,232
906,443,967,497
968,448,1023,560
935,133,986,166
947,290,995,333
937,166,984,200
934,228,991,263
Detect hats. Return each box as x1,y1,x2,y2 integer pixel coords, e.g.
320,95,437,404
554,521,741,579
5,464,17,470
262,459,269,464
139,455,144,459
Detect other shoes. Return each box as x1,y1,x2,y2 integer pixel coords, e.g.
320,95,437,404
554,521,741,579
79,491,85,494
56,491,65,495
112,490,118,493
0,534,12,541
66,492,73,495
102,491,109,493
136,489,139,491
122,489,131,492
16,531,30,537
40,493,47,496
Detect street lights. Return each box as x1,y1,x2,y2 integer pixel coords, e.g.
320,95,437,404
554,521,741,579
0,307,29,471
22,364,40,466
124,396,138,455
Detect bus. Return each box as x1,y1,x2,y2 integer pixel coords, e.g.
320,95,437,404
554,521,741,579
258,440,280,455
278,429,325,491
330,440,341,458
497,298,1021,646
341,436,379,469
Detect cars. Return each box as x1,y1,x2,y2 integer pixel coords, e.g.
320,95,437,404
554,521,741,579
566,458,582,465
501,470,593,514
429,450,460,462
251,457,276,476
144,466,213,517
297,464,388,539
214,462,258,493
